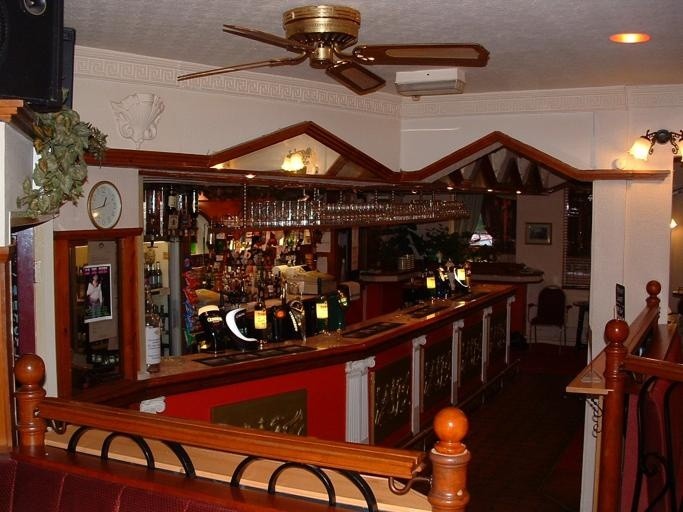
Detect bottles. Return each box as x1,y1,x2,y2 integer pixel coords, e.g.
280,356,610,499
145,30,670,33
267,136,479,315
144,183,197,248
143,259,165,373
205,217,315,302
253,298,268,334
310,277,329,335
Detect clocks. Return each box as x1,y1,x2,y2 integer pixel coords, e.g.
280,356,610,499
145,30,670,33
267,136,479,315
87,181,122,229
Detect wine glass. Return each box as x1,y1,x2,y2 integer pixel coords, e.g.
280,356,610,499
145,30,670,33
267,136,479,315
249,190,470,226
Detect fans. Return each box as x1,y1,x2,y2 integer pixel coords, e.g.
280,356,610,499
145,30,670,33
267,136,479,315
176,5,489,95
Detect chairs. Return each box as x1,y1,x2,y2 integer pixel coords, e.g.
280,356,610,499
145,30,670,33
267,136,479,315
527,285,572,349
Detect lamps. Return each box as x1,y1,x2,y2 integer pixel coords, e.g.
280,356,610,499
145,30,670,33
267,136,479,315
628,129,683,160
394,69,466,96
281,147,311,173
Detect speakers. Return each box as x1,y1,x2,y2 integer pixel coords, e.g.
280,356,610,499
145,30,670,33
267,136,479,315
0,0,64,106
26,27,75,113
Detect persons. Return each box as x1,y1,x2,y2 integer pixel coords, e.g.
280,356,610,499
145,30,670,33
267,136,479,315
75,283,86,341
85,271,102,319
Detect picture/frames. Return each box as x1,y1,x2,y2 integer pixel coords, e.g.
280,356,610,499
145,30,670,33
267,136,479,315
525,223,551,244
